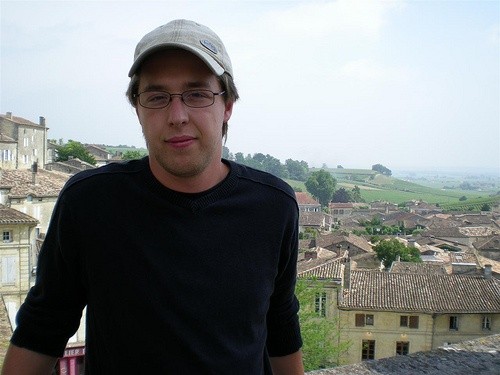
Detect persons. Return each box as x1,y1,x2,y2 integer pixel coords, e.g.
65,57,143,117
0,19,305,375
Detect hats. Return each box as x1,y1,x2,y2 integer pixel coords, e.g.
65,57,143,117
128,19,234,80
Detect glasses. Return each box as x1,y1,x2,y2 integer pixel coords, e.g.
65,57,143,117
132,89,226,109
